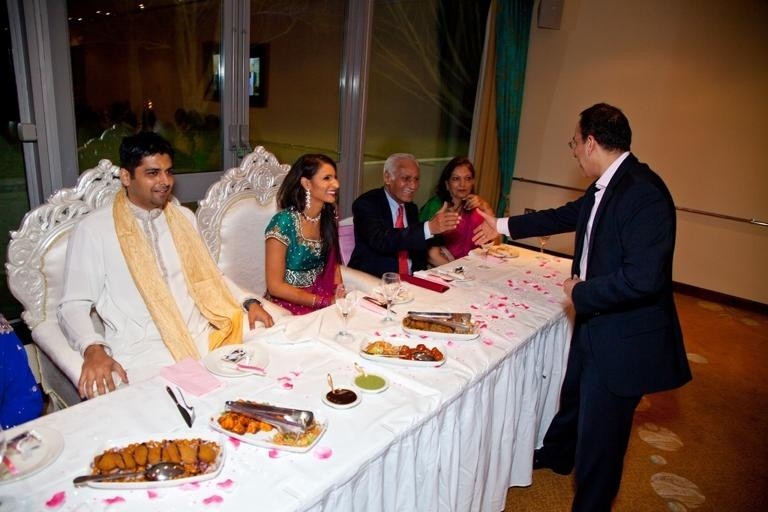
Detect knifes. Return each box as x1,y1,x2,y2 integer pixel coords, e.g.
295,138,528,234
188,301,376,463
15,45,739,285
166,385,194,427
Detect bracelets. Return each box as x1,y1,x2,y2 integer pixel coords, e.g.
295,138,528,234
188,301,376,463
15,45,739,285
244,298,260,310
312,293,318,306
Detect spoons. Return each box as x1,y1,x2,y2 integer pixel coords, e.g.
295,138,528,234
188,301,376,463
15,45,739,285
73,464,183,484
175,385,196,423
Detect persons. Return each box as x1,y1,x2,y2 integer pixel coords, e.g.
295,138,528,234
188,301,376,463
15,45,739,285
346,153,460,283
263,155,349,317
56,131,275,401
419,155,502,267
1,312,45,433
471,101,693,511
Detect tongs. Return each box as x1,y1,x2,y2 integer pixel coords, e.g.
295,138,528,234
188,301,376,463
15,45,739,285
225,399,313,436
405,310,475,331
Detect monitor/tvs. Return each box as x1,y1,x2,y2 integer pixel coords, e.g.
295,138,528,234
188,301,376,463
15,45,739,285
202,43,271,108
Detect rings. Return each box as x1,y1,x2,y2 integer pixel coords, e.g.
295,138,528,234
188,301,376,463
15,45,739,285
337,287,341,290
341,288,344,290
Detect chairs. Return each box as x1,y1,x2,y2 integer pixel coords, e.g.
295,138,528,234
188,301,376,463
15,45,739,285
335,213,357,270
194,143,391,325
73,118,139,181
4,157,292,412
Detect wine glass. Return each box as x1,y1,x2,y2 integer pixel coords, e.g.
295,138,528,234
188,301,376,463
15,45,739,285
335,284,357,338
380,271,401,325
536,236,550,262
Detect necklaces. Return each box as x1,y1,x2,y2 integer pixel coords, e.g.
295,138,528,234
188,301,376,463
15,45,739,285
302,212,322,223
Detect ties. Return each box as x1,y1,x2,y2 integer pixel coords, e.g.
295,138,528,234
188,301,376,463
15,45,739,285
394,205,411,282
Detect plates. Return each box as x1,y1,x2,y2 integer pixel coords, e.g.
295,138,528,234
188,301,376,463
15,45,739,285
357,375,387,394
373,286,414,303
401,315,483,341
208,408,327,451
325,387,362,410
0,424,62,484
206,344,270,378
85,442,225,490
430,244,518,281
361,334,447,366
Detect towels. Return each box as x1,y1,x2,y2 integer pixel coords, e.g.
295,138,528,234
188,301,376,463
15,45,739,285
343,268,452,319
157,355,224,400
